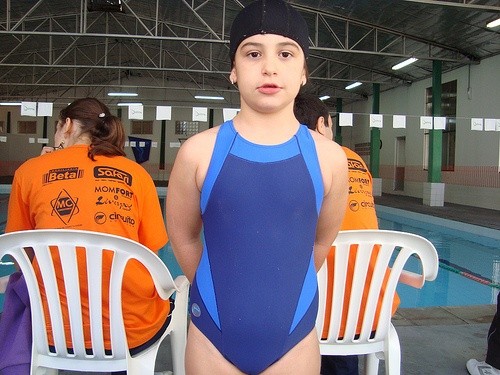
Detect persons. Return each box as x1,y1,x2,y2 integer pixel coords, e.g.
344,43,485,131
292,91,401,375
466,291,500,375
5,99,175,356
167,0,349,375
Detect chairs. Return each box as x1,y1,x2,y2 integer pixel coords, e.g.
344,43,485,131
0,229,191,375
316,228,439,375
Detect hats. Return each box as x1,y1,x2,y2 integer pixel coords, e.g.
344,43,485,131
230,0,310,68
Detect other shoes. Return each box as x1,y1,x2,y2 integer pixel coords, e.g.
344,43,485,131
466,359,500,375
